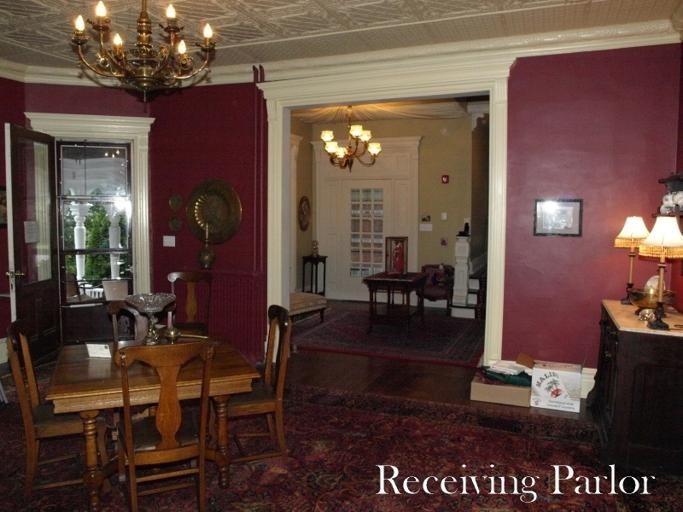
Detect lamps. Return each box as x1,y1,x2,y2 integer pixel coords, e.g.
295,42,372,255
71,0,215,103
320,105,383,174
614,216,683,331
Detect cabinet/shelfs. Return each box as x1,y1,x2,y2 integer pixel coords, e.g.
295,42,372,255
592,299,682,469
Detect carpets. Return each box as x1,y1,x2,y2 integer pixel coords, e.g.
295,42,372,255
0,381,683,512
289,308,485,369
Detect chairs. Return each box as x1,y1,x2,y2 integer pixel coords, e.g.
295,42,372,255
415,262,455,316
167,271,212,339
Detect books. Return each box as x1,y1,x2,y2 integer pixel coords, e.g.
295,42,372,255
487,358,525,376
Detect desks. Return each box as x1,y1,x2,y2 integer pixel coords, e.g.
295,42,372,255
362,272,429,335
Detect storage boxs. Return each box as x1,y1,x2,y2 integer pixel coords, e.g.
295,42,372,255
470,357,595,413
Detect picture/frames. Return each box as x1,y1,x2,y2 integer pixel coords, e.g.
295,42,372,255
533,198,583,237
